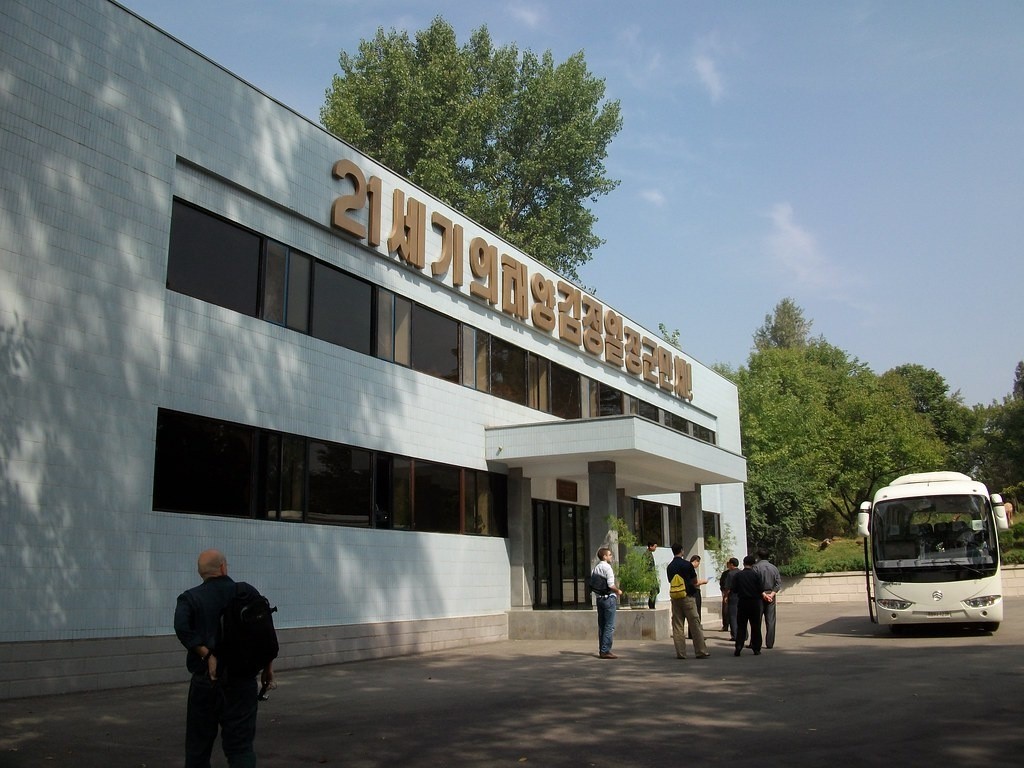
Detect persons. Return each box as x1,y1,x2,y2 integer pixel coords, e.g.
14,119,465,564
688,554,708,640
666,543,781,660
173,549,279,768
641,539,660,608
590,548,622,658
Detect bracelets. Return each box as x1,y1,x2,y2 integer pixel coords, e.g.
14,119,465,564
203,651,211,660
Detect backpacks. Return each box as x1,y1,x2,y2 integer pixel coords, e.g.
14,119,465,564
198,581,280,681
670,561,687,599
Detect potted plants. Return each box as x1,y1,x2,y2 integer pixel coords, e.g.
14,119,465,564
607,514,661,608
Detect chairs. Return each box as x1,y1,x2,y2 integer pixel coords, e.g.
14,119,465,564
883,521,969,560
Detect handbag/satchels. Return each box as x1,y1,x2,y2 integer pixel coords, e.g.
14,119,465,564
588,564,612,596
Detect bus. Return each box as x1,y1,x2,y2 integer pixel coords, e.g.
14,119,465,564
857,471,1010,635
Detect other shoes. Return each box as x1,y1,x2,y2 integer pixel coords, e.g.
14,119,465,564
719,627,728,631
729,638,736,641
755,650,761,655
734,646,741,656
697,652,710,658
745,644,752,648
600,651,617,659
